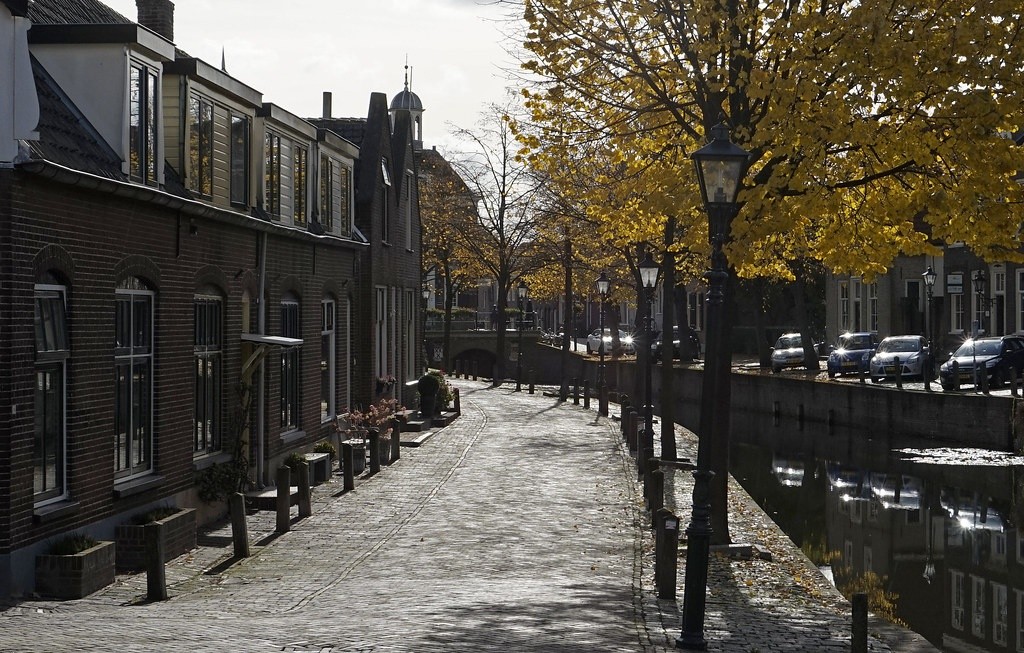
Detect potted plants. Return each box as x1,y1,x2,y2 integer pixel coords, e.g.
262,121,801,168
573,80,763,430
418,375,439,417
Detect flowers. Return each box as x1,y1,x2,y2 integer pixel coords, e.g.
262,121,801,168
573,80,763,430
369,399,407,438
333,409,368,446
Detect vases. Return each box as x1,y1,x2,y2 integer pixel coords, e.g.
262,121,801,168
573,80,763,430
343,444,367,473
378,435,390,465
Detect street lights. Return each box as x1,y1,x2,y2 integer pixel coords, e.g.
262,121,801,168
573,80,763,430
594,269,612,416
670,111,754,650
634,245,664,479
516,277,529,393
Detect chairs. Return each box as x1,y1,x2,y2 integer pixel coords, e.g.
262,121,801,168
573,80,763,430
336,412,379,470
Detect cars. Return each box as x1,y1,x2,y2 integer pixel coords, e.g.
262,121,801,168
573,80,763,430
938,333,1024,391
827,331,883,378
824,460,872,502
869,333,936,383
770,450,820,489
938,485,1016,533
587,328,635,355
650,325,702,361
768,332,821,372
870,474,941,516
